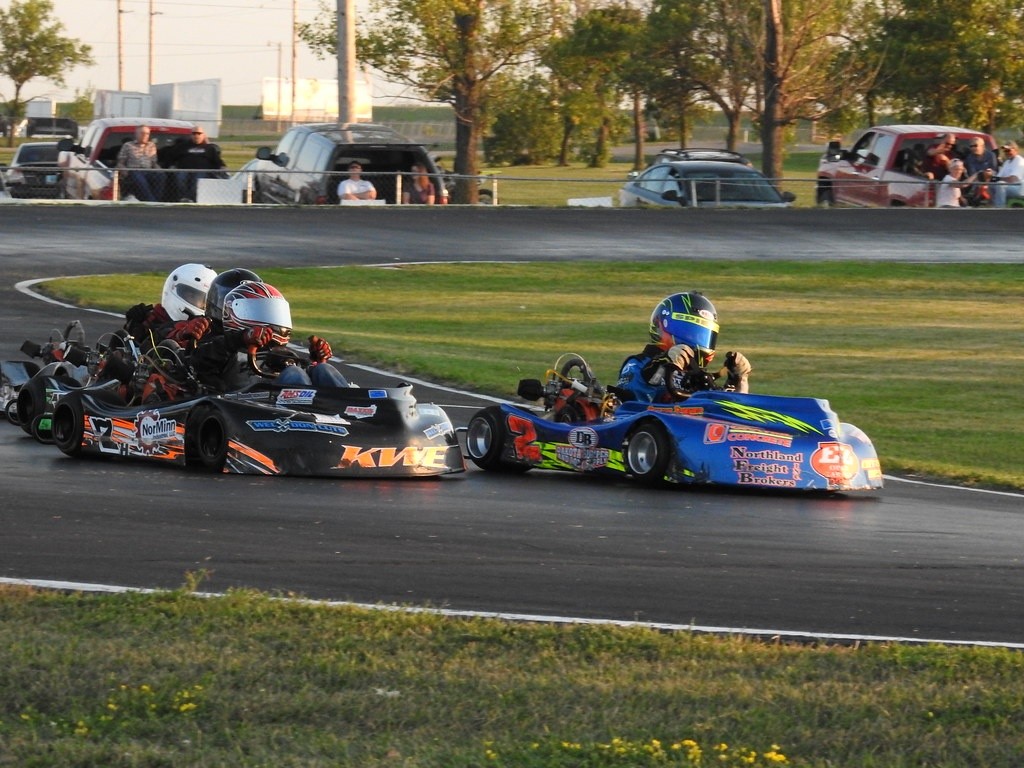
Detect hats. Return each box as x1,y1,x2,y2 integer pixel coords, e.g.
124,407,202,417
1001,140,1019,150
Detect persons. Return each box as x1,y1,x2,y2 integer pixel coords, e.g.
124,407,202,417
402,163,435,204
617,292,749,402
134,264,218,352
192,281,352,389
984,141,1024,208
337,161,377,200
191,268,263,387
935,158,983,207
117,125,172,202
169,126,226,202
965,137,997,183
923,133,956,179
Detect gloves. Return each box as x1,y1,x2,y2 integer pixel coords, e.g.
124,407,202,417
125,303,154,329
213,325,273,349
167,316,208,340
642,344,695,383
723,351,750,395
306,334,332,366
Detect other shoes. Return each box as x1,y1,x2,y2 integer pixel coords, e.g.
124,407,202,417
143,393,161,404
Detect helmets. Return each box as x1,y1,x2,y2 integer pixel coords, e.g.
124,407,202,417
223,283,292,348
648,290,718,363
160,263,217,323
205,269,263,322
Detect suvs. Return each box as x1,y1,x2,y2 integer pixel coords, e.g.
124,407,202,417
226,122,451,206
813,122,1001,209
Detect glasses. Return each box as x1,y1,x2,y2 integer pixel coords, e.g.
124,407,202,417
191,131,203,136
944,140,954,146
350,166,362,169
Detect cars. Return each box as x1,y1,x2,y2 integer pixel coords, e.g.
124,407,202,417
618,148,797,207
4,141,80,198
57,116,234,201
